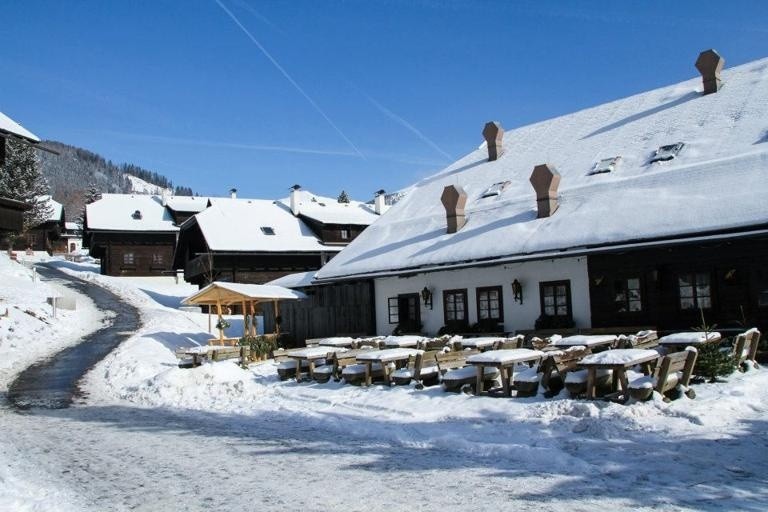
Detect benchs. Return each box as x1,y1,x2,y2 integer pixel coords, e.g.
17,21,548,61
273,327,760,401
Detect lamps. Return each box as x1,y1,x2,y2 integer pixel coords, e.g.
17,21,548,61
421,286,433,310
511,278,523,305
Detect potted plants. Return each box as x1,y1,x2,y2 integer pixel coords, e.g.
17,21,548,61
238,334,275,363
